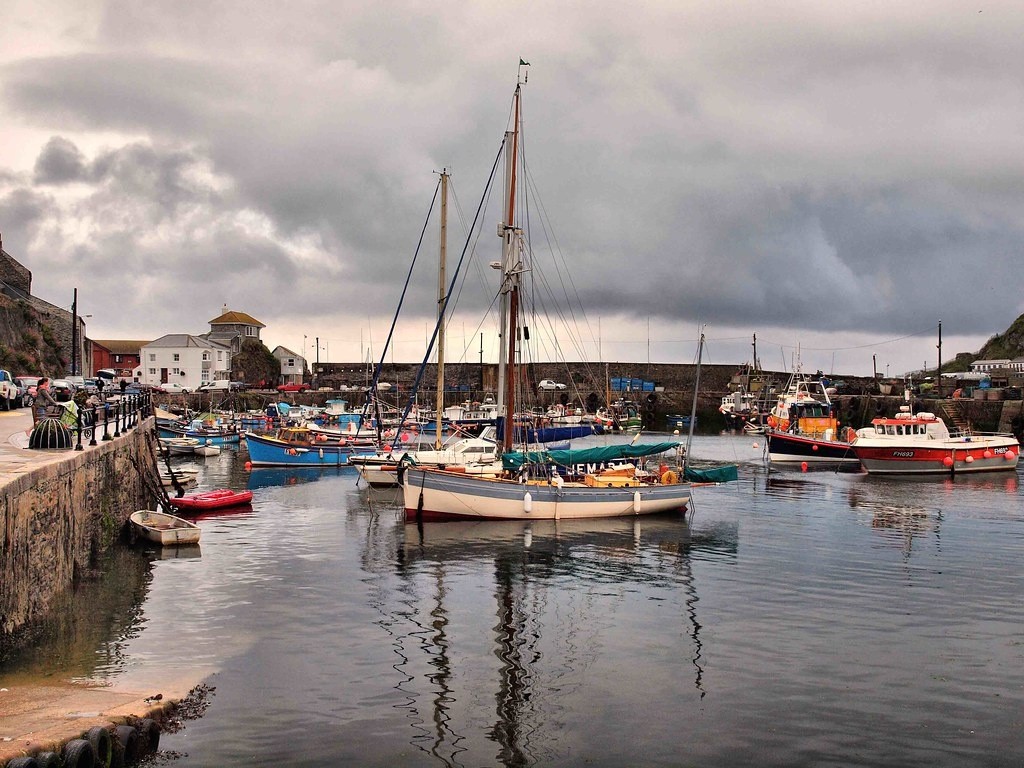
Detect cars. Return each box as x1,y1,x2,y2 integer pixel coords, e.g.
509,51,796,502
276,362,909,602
0,370,20,412
231,380,245,394
538,380,566,392
15,375,115,407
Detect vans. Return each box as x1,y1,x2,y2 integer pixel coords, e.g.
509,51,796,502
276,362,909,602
199,380,231,394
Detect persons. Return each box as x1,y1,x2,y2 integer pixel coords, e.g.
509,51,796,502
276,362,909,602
96,376,105,391
35,377,57,420
268,379,274,392
261,379,265,391
120,378,126,395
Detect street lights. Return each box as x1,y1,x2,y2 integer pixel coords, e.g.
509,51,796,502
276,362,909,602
71,314,94,375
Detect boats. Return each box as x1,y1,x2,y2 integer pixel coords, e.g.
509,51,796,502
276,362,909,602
845,406,1019,477
129,508,202,548
170,488,253,514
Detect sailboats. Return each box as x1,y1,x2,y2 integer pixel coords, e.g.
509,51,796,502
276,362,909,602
154,57,860,525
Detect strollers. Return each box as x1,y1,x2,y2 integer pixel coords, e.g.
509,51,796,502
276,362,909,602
52,400,92,439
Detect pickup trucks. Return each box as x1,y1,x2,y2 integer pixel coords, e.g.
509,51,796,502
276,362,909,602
132,384,167,396
276,381,310,393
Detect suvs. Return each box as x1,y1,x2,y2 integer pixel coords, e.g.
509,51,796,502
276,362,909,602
160,383,193,395
